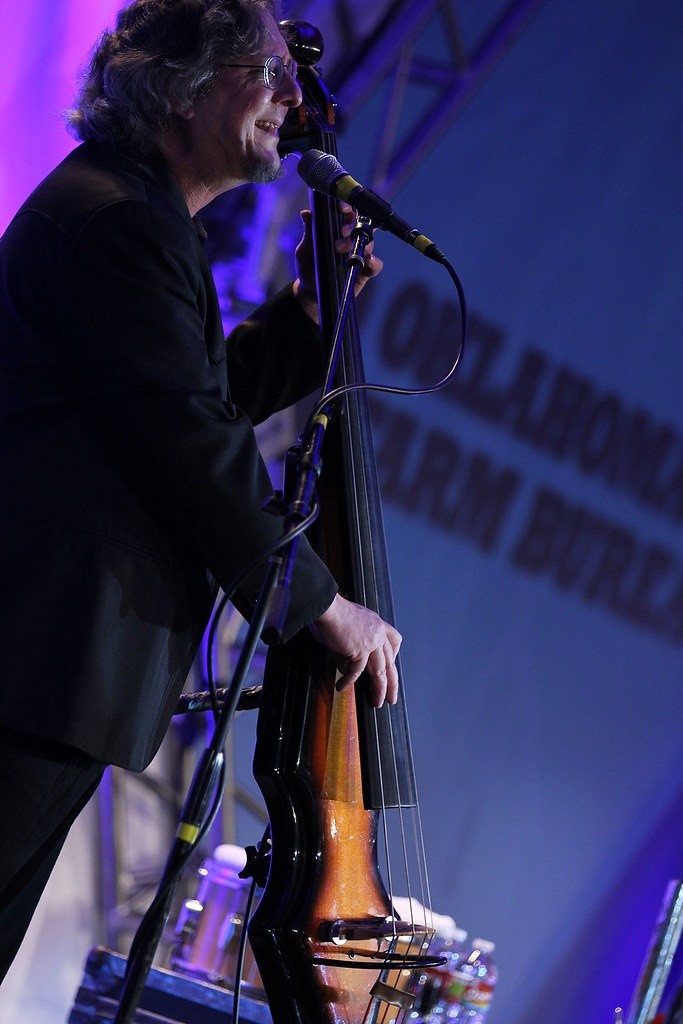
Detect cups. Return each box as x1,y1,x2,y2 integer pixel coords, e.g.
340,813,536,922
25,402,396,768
176,842,248,973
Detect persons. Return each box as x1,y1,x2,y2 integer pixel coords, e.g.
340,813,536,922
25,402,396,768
0,1,405,993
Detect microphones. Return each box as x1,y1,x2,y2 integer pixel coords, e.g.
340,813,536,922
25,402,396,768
296,150,447,263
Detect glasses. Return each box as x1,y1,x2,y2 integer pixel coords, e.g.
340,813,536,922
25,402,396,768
221,55,297,90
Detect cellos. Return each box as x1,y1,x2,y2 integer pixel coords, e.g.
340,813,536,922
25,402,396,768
242,16,449,1024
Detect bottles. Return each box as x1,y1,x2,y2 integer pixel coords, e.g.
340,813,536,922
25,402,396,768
406,928,499,1024
